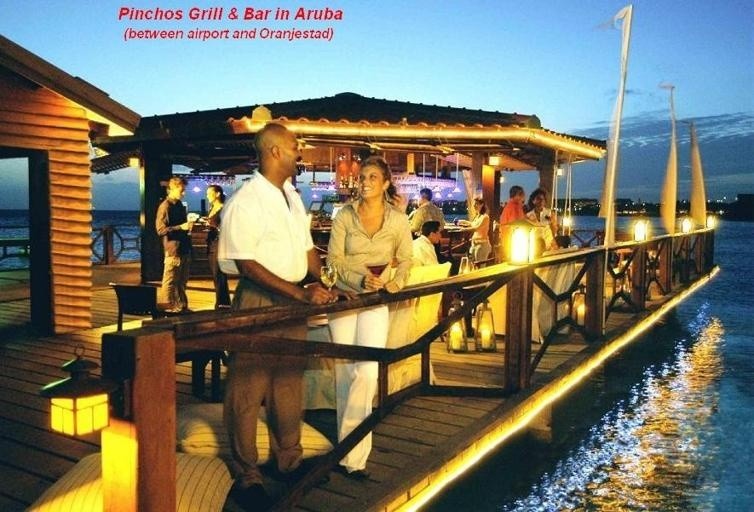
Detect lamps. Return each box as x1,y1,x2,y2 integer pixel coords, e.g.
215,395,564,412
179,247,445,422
561,212,715,242
40,344,119,438
502,218,545,265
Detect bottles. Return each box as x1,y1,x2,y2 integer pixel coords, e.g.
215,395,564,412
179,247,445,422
559,219,570,237
338,172,359,189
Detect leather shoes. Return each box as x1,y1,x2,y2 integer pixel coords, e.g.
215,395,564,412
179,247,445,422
341,466,371,480
239,485,274,510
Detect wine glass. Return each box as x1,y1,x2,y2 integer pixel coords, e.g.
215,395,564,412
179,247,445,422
365,262,388,295
186,216,193,235
319,263,338,304
201,217,209,232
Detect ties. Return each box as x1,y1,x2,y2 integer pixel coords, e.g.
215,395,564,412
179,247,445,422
289,464,330,485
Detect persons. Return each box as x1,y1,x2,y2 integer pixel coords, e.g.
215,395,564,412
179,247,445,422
412,221,442,266
410,187,445,264
199,184,232,310
459,197,491,268
154,176,194,314
499,185,526,247
549,235,570,251
215,124,358,489
325,156,414,482
525,188,558,250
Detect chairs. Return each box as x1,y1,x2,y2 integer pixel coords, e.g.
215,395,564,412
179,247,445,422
108,221,501,401
